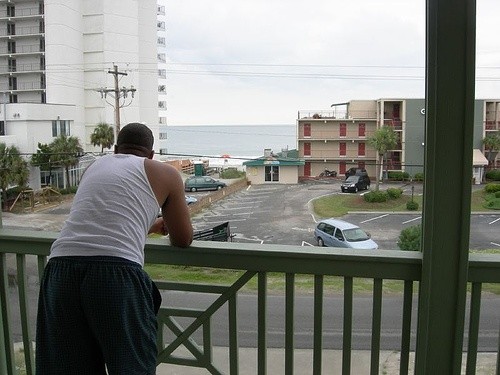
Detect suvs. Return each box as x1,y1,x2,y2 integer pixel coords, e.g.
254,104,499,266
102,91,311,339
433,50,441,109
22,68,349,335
340,174,370,192
184,175,228,192
314,219,379,252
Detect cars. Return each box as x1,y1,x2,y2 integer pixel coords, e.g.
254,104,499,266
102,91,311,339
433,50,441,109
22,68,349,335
344,166,367,177
185,194,197,206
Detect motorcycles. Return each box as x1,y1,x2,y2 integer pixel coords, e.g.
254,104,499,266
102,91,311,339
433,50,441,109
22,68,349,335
319,168,336,176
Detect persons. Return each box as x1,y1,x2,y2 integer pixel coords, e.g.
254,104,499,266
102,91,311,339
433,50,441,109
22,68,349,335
34,122,192,375
